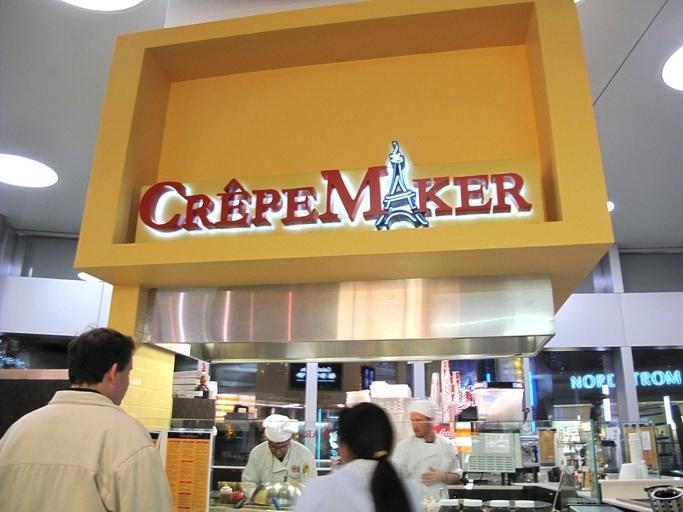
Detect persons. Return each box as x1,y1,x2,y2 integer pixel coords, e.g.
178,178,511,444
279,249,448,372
391,400,463,512
239,414,319,500
290,401,425,511
0,327,174,511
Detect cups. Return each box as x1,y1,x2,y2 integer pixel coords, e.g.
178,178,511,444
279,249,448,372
429,360,465,421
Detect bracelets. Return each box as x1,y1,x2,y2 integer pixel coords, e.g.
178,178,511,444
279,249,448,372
444,472,448,482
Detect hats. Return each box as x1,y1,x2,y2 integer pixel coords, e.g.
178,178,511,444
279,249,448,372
408,399,437,419
261,414,294,444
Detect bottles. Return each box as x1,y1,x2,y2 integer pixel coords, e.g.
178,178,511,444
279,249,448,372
220,484,234,505
194,369,212,400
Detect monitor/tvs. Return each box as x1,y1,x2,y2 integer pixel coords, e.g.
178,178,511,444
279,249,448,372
288,362,343,391
